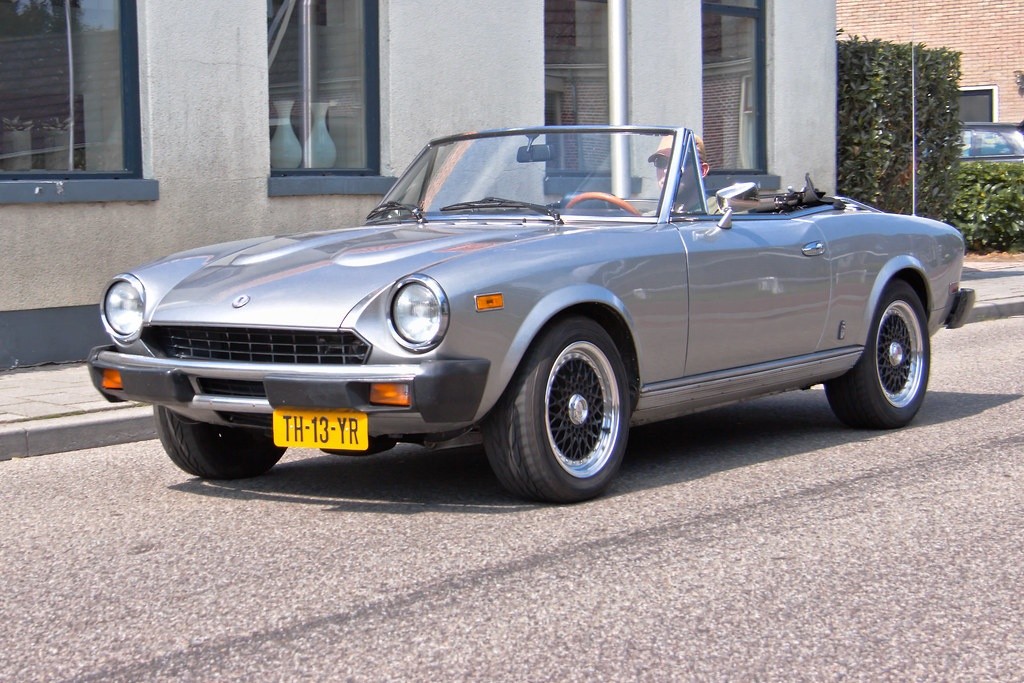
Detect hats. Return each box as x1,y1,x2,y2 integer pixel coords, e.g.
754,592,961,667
648,134,706,163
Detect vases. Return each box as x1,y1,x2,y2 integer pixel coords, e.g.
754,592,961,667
312,102,337,168
269,100,302,170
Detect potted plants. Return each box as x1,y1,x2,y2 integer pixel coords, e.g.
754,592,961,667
39,116,71,170
1,115,35,172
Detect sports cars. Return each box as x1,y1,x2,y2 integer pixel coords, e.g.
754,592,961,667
86,122,978,505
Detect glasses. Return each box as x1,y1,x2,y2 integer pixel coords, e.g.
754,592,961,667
654,156,668,167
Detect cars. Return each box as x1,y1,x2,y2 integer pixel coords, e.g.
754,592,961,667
915,120,1024,163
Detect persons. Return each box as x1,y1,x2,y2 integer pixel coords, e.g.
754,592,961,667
641,131,722,217
